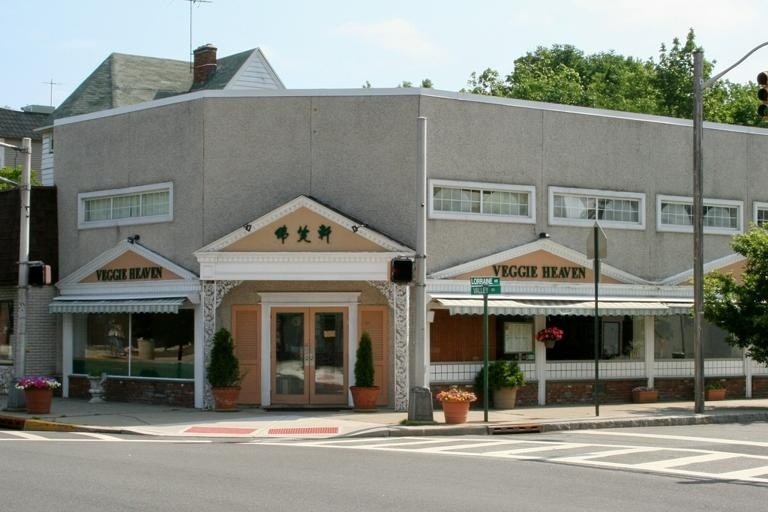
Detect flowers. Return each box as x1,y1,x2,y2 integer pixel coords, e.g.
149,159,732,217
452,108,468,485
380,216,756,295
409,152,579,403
533,327,564,344
14,373,60,391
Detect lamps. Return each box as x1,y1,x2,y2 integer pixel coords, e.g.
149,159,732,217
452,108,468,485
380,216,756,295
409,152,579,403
127,234,141,245
539,233,549,238
351,224,366,233
242,223,251,232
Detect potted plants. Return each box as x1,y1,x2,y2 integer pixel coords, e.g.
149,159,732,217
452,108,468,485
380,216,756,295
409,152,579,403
207,328,250,411
349,333,381,411
631,385,660,403
705,383,728,401
474,358,526,410
436,387,478,423
84,365,109,405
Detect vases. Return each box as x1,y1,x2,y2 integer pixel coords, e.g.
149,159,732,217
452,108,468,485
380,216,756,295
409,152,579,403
542,338,557,350
20,386,54,414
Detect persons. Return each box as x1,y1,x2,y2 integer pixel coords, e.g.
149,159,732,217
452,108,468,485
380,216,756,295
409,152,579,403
105,317,125,359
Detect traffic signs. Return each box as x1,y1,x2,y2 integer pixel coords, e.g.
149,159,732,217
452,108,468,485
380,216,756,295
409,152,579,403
471,285,502,295
470,276,501,286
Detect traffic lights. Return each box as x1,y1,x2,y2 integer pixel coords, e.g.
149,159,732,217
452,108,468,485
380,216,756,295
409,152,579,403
28,264,51,286
390,258,414,283
